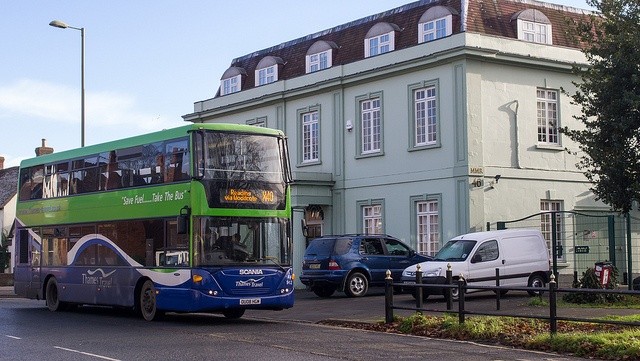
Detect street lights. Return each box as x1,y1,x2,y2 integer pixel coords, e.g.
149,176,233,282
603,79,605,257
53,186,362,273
48,21,84,146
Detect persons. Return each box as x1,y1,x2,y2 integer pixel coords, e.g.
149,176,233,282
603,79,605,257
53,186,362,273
227,232,248,261
150,153,164,183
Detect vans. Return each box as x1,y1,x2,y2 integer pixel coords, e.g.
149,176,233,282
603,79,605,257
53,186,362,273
401,229,552,302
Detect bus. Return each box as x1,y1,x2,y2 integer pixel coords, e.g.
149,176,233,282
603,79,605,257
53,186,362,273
13,123,295,322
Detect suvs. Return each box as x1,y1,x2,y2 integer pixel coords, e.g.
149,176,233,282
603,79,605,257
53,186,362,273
299,234,434,298
